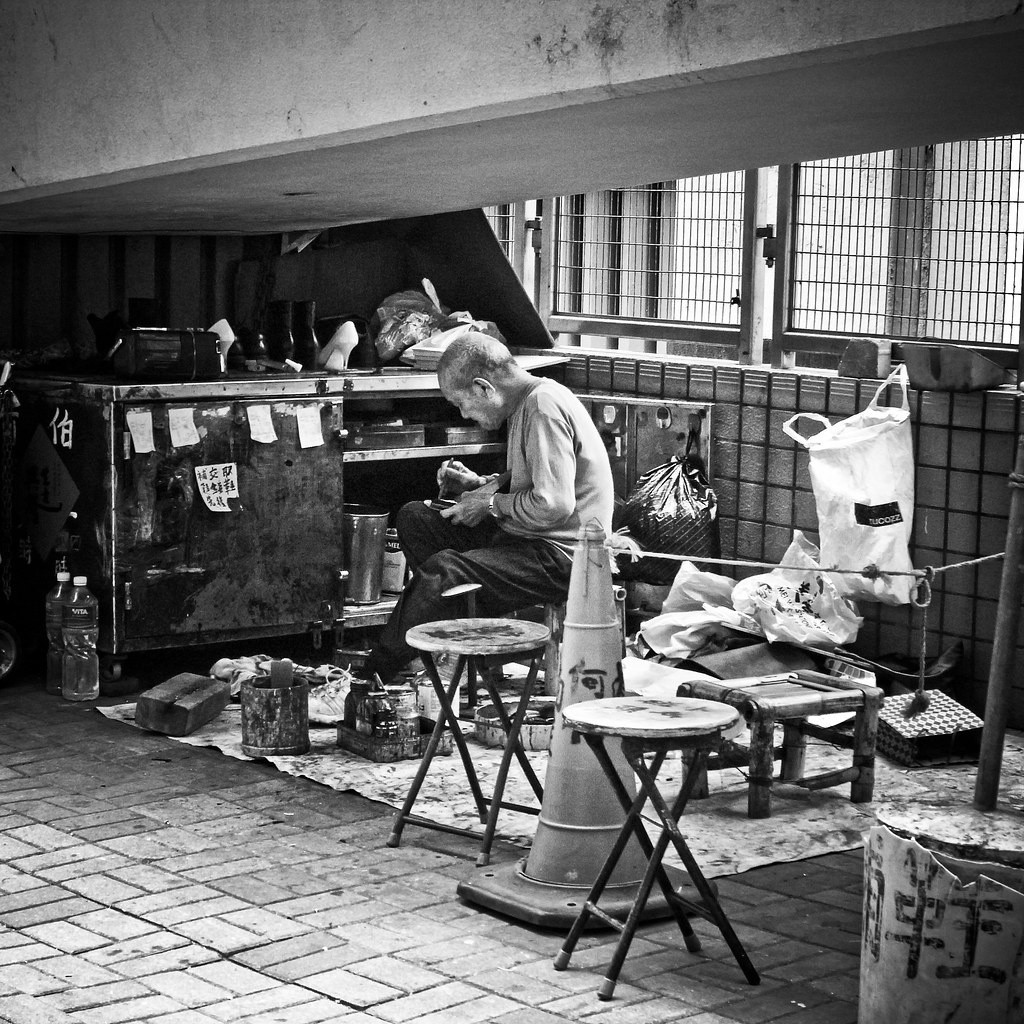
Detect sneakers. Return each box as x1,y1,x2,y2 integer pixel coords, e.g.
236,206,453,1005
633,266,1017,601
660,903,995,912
308,664,374,722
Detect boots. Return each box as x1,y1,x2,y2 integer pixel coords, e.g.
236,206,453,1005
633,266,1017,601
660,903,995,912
293,300,318,371
266,301,293,361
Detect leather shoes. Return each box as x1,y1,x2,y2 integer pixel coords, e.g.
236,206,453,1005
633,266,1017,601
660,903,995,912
227,341,245,364
241,334,268,361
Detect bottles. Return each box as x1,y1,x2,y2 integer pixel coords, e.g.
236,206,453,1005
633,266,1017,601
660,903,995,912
59,574,101,702
44,571,74,696
343,679,424,740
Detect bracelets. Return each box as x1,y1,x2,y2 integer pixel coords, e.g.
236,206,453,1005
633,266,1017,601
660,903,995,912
488,492,500,519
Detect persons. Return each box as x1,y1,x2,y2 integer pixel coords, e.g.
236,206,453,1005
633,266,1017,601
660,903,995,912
306,331,617,727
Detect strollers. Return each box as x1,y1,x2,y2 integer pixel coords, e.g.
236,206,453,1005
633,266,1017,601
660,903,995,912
0,359,23,691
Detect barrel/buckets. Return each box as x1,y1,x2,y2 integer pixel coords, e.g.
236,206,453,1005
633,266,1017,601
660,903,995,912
340,502,390,606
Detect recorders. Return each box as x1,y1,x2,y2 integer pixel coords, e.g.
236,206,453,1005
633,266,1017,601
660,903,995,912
105,326,222,383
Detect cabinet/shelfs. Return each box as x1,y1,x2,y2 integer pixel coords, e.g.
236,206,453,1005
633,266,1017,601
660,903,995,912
0,359,563,683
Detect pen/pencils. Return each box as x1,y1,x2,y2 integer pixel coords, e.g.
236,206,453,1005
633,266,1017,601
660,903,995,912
439,457,453,500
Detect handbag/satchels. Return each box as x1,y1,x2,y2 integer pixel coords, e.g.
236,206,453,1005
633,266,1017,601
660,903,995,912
781,364,919,605
878,689,983,767
733,527,865,645
628,430,721,578
375,278,485,363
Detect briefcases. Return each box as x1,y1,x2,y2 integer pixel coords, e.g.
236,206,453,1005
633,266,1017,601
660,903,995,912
98,327,222,382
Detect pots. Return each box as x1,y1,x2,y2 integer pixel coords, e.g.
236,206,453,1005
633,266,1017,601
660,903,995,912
104,327,221,383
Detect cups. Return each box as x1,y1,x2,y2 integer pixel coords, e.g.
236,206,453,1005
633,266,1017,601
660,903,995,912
239,674,311,755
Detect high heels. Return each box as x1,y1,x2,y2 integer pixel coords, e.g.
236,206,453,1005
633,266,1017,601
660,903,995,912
317,320,359,371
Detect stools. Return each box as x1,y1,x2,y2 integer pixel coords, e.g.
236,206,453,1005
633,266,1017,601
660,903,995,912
676,669,884,820
481,580,626,696
385,619,552,866
555,695,760,1001
